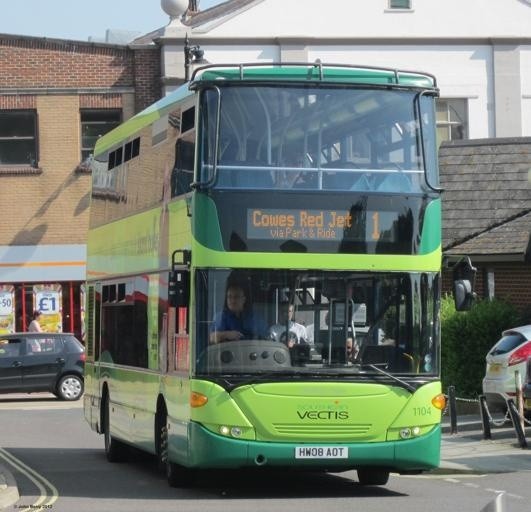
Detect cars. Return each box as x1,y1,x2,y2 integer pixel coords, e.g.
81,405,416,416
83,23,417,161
481,323,531,428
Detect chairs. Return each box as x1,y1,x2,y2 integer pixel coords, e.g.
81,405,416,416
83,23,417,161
205,161,274,187
293,161,411,190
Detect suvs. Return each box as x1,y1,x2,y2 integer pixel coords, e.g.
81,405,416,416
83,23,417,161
1,332,86,402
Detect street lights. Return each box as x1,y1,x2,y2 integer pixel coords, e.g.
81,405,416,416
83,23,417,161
182,32,211,84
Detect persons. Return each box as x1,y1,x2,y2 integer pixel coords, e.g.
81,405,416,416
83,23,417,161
265,303,308,347
28,310,43,352
345,337,359,365
208,286,273,341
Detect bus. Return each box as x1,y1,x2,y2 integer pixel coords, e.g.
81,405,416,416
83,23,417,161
81,62,449,488
